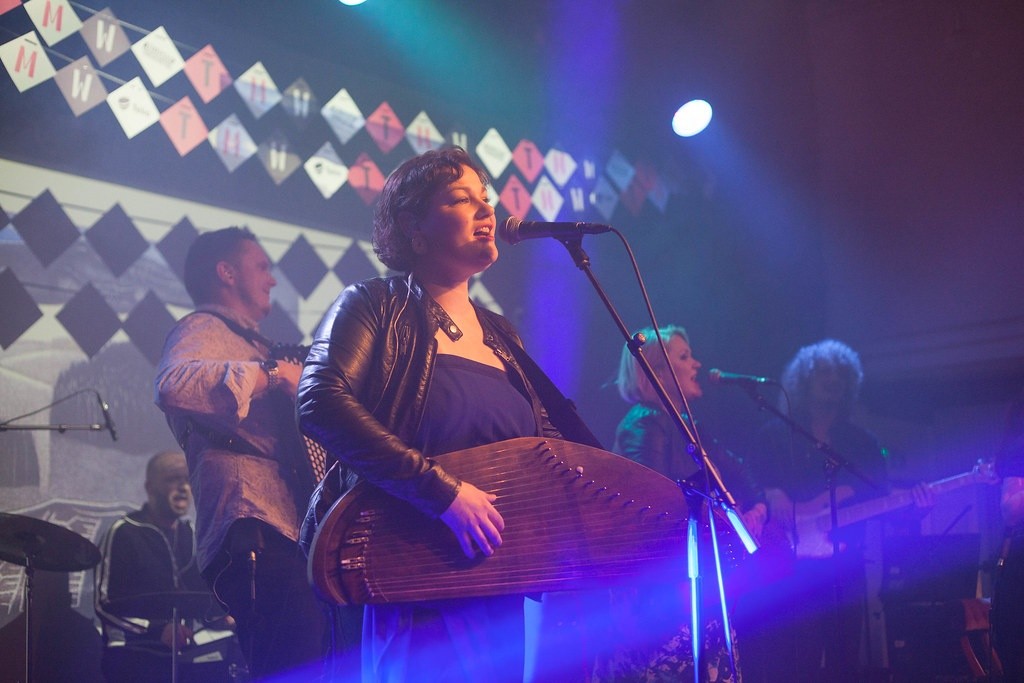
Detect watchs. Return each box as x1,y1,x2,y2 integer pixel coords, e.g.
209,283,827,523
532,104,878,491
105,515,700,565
259,359,279,398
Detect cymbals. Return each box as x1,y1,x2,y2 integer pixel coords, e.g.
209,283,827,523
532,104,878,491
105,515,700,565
0,512,103,574
100,590,218,621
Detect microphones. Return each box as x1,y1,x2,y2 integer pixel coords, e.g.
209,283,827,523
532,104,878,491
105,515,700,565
96,392,120,441
709,367,775,386
498,215,611,244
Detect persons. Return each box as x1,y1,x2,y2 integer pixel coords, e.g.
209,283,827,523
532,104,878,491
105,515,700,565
153,227,338,683
612,325,769,683
93,450,252,683
999,396,1024,683
295,144,607,683
744,338,939,683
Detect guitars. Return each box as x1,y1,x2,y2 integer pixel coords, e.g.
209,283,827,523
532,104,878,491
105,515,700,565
762,457,1002,563
